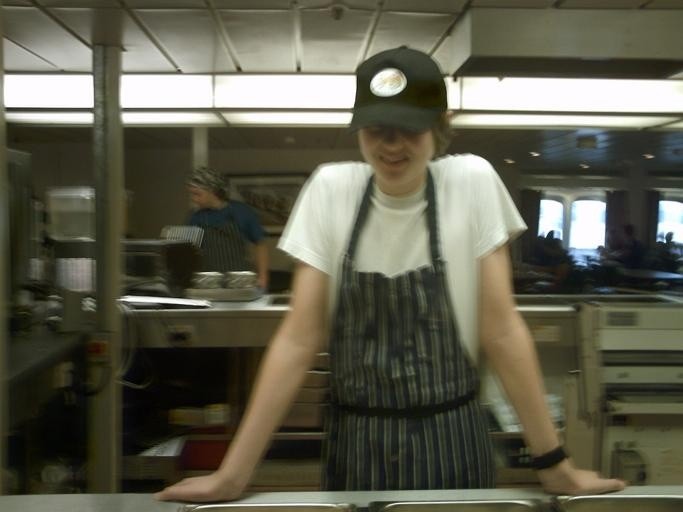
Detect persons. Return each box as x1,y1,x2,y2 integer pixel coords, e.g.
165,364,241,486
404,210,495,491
152,46,629,502
610,224,643,268
183,166,271,293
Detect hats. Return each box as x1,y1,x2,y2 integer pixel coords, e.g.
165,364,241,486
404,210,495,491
188,167,224,191
347,44,447,135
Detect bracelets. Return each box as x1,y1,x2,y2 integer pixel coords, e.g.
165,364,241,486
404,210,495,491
532,446,568,470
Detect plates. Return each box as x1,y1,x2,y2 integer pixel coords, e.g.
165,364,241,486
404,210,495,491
182,288,262,301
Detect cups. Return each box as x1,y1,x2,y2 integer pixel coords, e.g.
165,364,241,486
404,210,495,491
193,271,257,289
